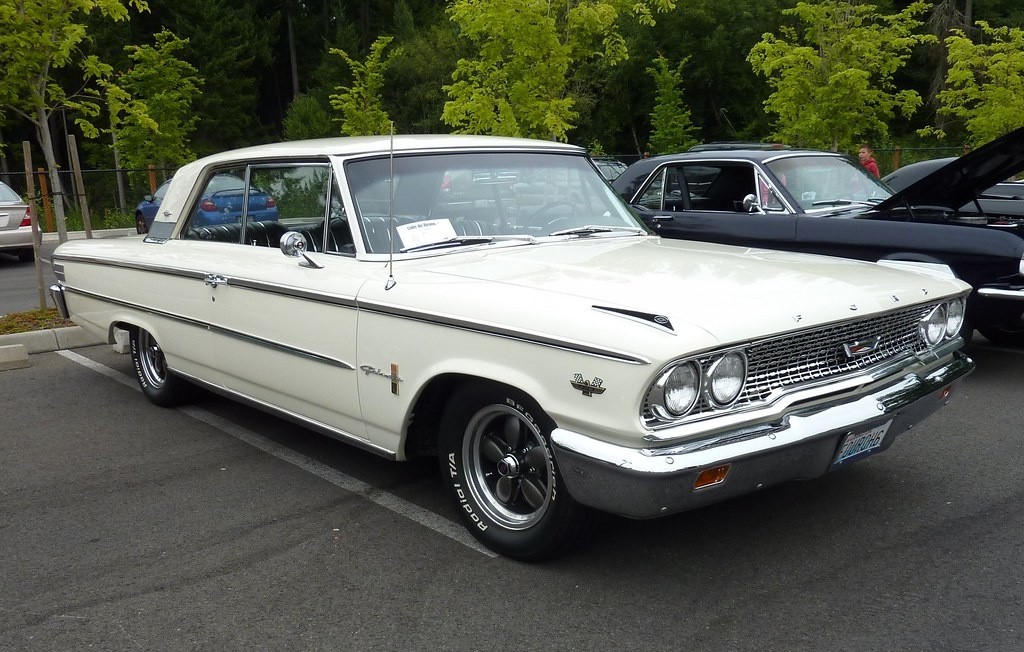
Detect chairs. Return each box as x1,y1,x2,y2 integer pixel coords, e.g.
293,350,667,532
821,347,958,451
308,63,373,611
452,219,491,237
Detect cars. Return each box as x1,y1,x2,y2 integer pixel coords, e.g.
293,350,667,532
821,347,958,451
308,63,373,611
608,126,1023,358
50,132,977,560
134,169,280,236
0,180,44,263
345,139,1024,257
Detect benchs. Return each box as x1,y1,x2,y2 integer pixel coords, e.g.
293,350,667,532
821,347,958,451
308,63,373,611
190,217,417,253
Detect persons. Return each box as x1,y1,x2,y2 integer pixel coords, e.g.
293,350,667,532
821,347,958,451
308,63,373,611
858,145,879,178
